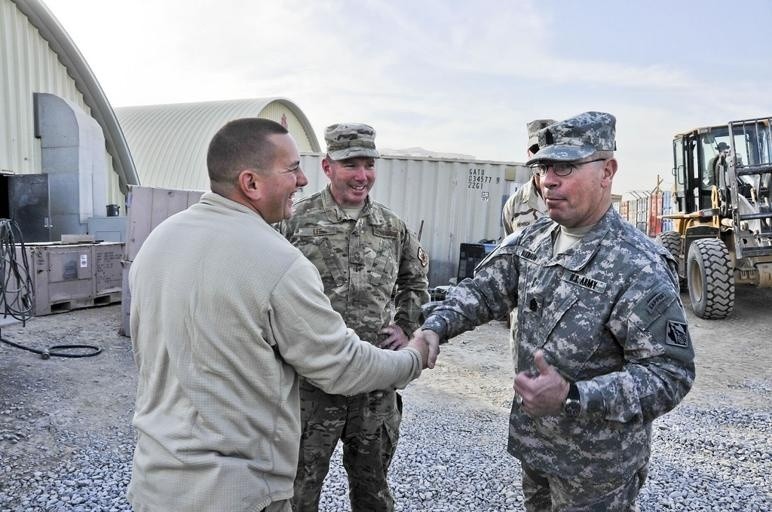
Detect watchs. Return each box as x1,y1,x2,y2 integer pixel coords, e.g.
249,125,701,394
563,383,579,420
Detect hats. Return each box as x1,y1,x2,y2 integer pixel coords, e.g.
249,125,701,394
525,111,616,161
525,119,559,151
715,142,729,149
324,124,379,161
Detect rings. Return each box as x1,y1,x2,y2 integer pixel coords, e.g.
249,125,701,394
515,395,525,407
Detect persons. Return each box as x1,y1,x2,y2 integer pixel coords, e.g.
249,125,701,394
501,116,558,385
269,120,430,510
129,118,438,510
703,141,739,189
418,112,696,510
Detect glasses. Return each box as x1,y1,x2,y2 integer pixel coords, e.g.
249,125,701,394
530,159,607,176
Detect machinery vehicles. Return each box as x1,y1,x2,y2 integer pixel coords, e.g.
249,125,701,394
651,114,770,322
